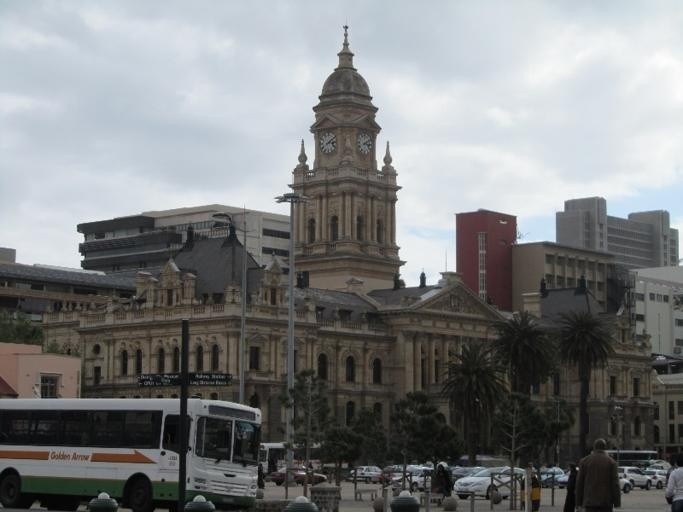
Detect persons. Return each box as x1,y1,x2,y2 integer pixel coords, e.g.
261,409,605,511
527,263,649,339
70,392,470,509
575,438,621,512
563,465,577,512
527,461,538,479
433,464,452,496
666,455,677,486
665,453,683,512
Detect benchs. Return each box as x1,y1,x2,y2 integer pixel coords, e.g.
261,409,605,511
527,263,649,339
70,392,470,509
355,489,377,501
420,493,444,505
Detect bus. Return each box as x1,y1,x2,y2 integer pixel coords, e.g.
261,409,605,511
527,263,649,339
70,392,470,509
0,398,261,512
589,450,659,466
258,442,326,474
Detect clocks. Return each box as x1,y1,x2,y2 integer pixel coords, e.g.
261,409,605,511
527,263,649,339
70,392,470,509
319,132,337,155
356,133,373,155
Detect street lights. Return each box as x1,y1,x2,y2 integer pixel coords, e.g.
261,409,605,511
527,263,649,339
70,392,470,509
281,193,309,485
